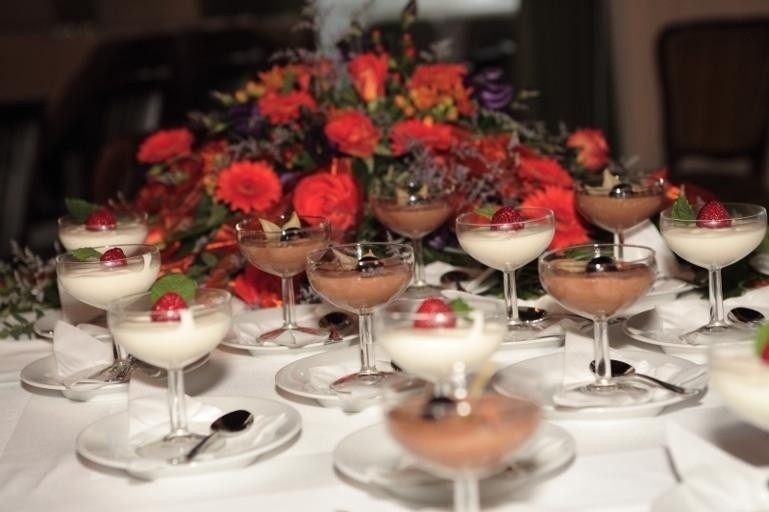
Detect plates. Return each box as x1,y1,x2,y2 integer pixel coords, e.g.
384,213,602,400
32,310,110,343
491,348,711,421
620,295,767,355
77,396,305,481
631,255,710,298
341,416,581,507
271,340,429,410
220,304,360,356
20,338,210,402
402,251,502,299
500,301,597,354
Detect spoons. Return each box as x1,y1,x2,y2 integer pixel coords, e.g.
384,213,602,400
505,305,594,327
169,409,254,465
319,310,352,344
438,266,470,293
588,357,705,401
726,305,766,334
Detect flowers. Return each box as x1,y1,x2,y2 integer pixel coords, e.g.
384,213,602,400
1,0,670,339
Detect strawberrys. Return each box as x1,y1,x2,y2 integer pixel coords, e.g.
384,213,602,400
695,198,732,229
151,292,187,322
85,208,117,232
414,299,456,327
490,205,524,231
100,247,127,268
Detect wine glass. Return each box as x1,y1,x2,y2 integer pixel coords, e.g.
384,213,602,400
372,180,458,300
658,199,768,346
306,244,409,395
236,212,332,347
538,245,659,408
55,244,163,383
573,172,665,263
107,285,235,460
375,345,552,512
54,209,152,261
452,208,557,342
369,296,510,399
